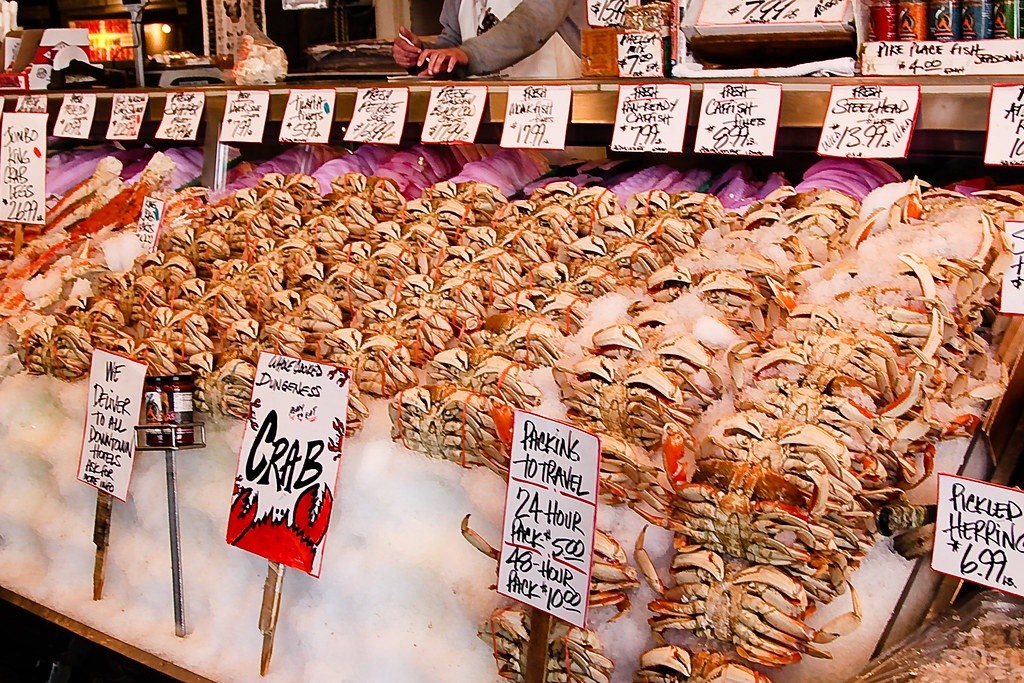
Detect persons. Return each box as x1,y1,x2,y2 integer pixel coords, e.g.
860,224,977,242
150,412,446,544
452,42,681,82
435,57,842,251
392,1,582,78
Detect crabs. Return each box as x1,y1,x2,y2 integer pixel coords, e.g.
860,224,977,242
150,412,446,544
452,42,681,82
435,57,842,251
2,151,1024,683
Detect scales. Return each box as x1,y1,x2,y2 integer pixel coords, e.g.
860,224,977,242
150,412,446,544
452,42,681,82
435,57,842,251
120,0,237,88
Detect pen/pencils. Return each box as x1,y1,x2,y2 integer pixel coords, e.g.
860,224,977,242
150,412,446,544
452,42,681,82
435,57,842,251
398,31,431,63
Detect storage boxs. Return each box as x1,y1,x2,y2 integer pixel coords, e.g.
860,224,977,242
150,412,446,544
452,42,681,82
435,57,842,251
0,27,106,95
